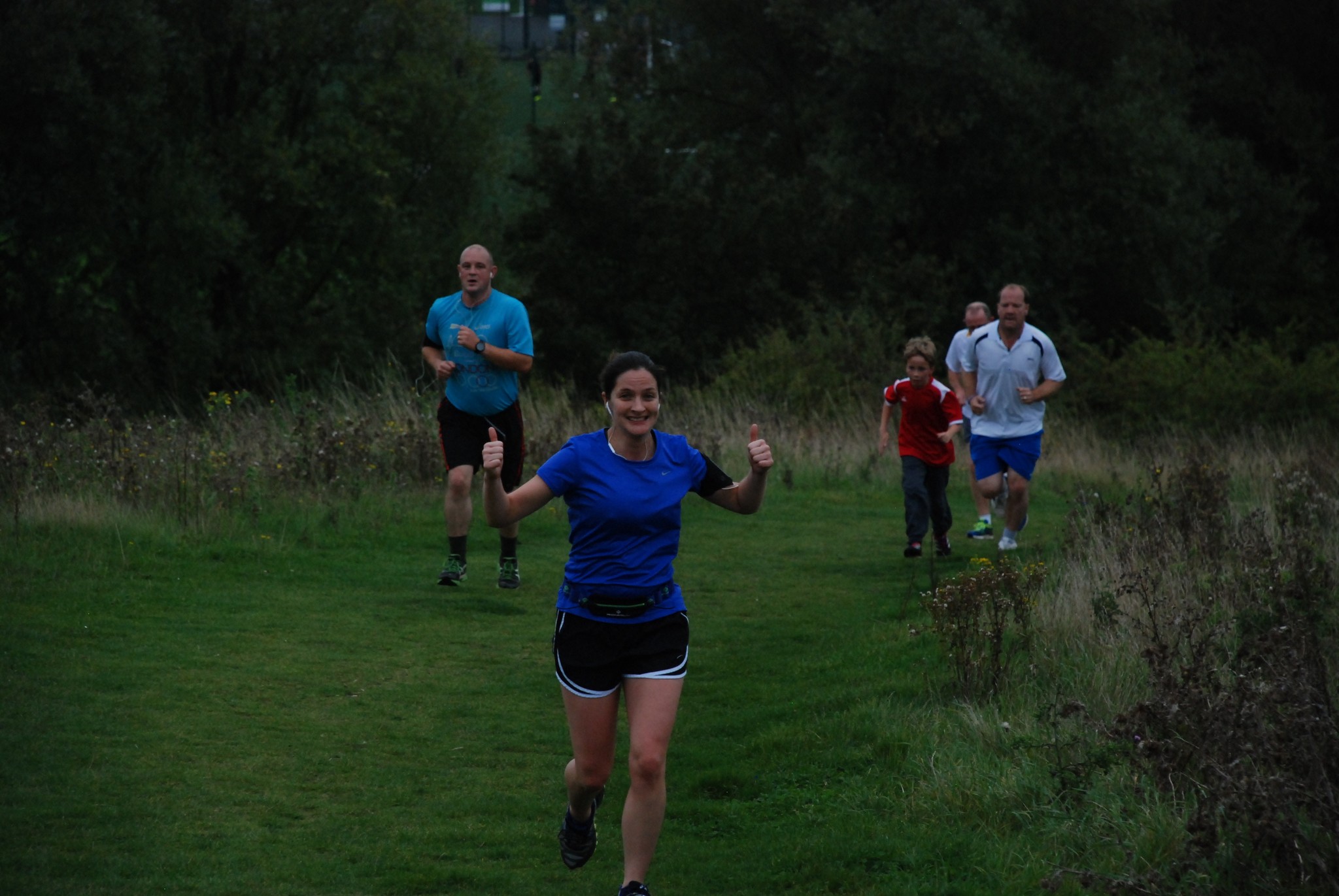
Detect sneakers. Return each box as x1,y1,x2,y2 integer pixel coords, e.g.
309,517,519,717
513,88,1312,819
991,475,1011,518
905,542,921,557
559,783,605,868
618,880,650,896
1018,511,1028,531
499,554,520,588
969,519,994,539
998,535,1019,550
437,554,469,588
934,531,952,557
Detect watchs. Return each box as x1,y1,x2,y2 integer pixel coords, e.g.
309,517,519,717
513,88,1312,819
475,340,487,353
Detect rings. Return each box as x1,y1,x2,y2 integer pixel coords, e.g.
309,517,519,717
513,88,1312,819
1024,396,1027,400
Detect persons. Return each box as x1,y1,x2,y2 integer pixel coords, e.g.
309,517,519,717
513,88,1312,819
483,349,773,896
879,281,1066,558
423,243,535,589
